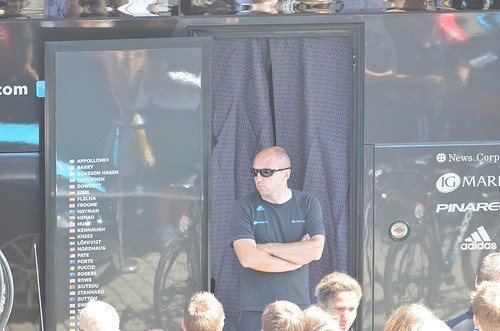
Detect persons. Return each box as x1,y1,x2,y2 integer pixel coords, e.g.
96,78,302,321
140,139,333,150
182,291,225,331
261,300,305,331
230,146,326,331
303,305,340,331
385,303,452,331
78,299,119,331
469,280,500,331
315,272,363,331
474,253,500,331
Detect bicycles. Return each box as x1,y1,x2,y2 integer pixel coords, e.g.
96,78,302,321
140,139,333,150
382,154,499,321
153,160,201,331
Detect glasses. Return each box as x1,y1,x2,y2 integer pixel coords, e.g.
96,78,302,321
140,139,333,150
250,168,290,177
